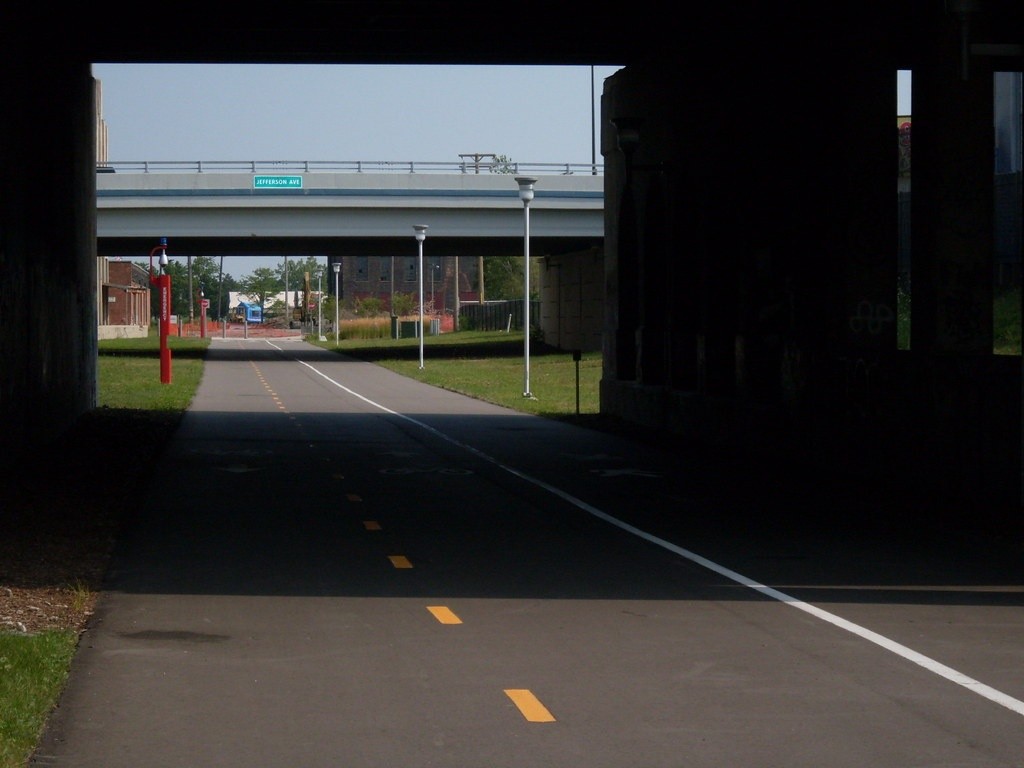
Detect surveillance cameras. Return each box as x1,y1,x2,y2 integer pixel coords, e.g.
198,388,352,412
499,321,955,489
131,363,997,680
201,291,205,297
159,255,168,267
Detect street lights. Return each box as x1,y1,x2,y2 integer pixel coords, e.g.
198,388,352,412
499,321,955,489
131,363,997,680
150,237,172,384
515,176,539,401
331,262,342,346
316,267,324,342
413,224,429,371
199,280,207,339
